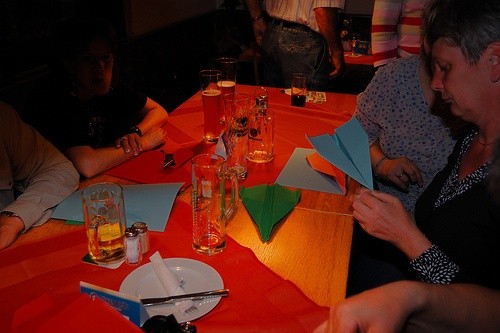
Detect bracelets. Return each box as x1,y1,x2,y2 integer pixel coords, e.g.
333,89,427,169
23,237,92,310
373,155,387,168
252,13,264,22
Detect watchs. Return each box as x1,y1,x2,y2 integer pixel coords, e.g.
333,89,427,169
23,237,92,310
1,211,18,217
125,125,142,138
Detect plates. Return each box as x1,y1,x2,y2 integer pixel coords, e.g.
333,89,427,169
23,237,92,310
119,258,224,324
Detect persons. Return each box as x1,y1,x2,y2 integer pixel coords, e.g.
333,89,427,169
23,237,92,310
314,0,500,333
0,28,169,253
246,0,345,91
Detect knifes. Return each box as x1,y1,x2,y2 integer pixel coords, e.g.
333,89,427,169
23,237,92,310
141,289,229,304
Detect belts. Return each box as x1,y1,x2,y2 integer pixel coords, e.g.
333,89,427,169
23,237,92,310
265,16,307,31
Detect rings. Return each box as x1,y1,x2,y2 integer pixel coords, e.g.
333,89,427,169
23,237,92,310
121,137,127,141
397,172,403,179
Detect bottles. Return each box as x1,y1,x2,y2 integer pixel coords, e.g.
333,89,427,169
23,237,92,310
351,37,356,56
125,228,138,263
256,95,268,116
131,222,149,259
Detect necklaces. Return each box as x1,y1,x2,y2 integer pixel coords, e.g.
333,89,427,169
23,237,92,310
476,139,499,146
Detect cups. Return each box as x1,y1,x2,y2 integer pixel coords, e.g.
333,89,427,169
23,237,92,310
291,73,306,107
217,93,260,181
192,154,240,255
200,69,223,141
82,182,127,264
217,58,238,122
246,108,274,163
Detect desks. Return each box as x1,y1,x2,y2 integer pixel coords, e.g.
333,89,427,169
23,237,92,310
0,84,361,333
